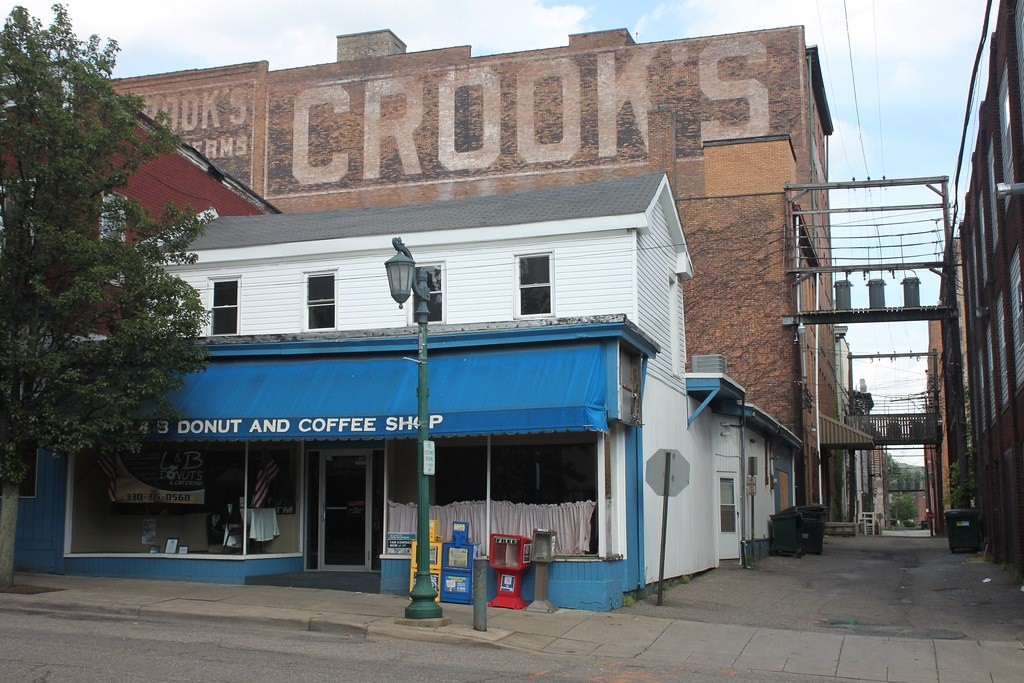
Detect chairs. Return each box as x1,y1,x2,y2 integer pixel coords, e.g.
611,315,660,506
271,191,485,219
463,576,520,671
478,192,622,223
224,519,243,549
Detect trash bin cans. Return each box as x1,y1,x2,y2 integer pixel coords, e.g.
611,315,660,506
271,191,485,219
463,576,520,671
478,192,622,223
798,506,829,554
921,520,928,530
768,506,803,557
944,509,980,554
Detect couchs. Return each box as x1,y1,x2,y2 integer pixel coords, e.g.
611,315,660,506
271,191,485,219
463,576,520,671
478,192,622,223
206,512,225,546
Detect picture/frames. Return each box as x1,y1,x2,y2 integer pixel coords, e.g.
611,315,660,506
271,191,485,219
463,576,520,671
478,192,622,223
149,537,189,554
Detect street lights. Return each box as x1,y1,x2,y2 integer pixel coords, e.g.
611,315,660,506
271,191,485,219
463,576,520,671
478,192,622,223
385,236,450,620
